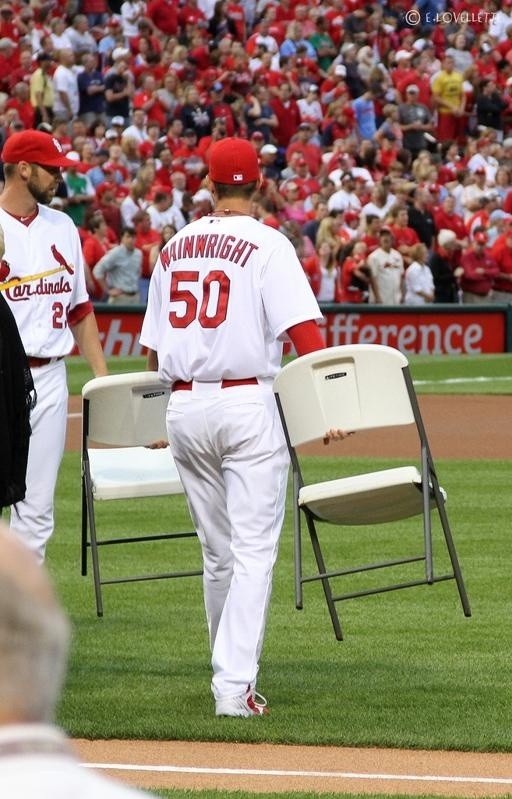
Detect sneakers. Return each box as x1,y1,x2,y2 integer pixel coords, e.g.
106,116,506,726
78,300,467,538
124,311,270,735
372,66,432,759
212,686,272,720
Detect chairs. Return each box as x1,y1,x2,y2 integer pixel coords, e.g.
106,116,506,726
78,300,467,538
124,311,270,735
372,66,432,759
272,344,474,643
79,370,204,620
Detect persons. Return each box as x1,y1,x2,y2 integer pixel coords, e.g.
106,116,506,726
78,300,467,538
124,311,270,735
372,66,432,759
103,56,131,127
29,52,55,132
31,0,128,57
458,226,499,304
0,0,31,130
402,243,435,305
139,136,351,719
78,53,106,128
54,48,80,125
94,227,142,307
337,242,369,305
0,129,113,565
221,105,512,248
365,227,404,306
491,229,512,302
132,1,509,104
0,519,158,799
148,225,178,276
1,108,217,233
425,228,459,305
302,241,342,305
82,215,119,301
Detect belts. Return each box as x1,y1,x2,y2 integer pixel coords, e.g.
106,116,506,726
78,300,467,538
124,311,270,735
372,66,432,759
168,375,263,391
29,353,66,369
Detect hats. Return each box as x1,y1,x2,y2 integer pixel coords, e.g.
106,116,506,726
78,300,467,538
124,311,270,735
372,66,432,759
473,165,487,176
111,46,129,60
406,84,419,94
334,64,347,77
309,83,319,92
344,212,360,223
206,136,261,185
379,225,393,234
110,115,125,127
476,136,491,150
260,144,278,155
104,128,119,139
36,51,53,62
474,231,489,245
0,128,80,168
394,50,414,62
251,130,264,142
37,121,53,133
101,161,116,173
480,40,493,54
